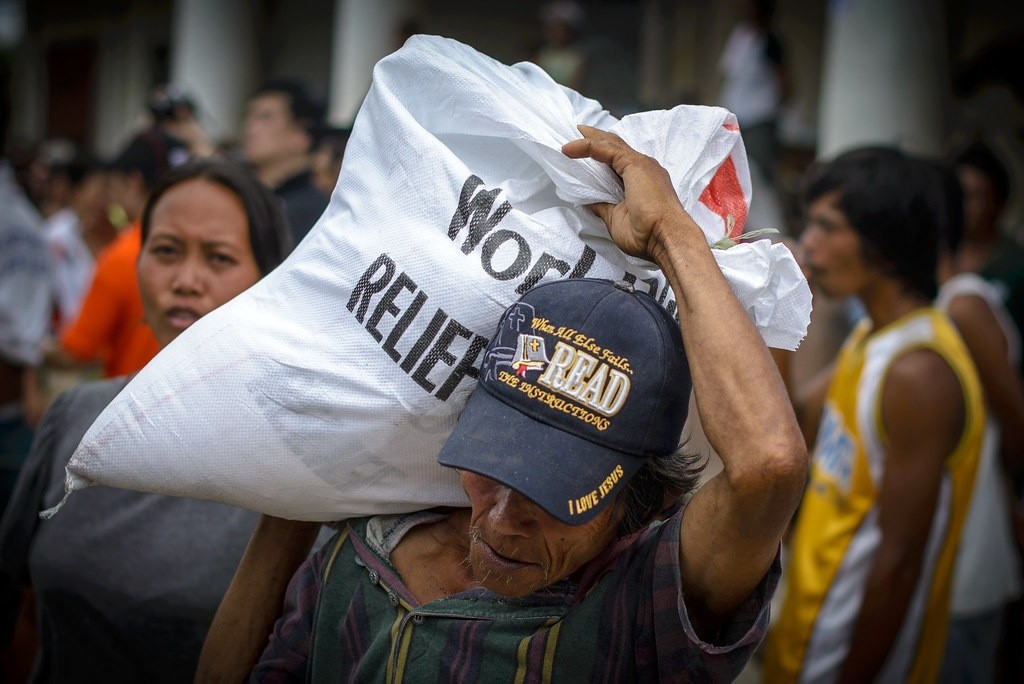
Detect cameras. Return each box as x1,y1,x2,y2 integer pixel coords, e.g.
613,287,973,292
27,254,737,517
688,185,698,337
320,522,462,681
150,91,195,126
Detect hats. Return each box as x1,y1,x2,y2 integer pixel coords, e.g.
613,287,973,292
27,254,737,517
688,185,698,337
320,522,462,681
437,278,692,526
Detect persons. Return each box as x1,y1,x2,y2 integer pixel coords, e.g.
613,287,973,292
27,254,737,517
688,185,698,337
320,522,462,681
0,82,1023,684
718,0,786,171
527,0,587,98
